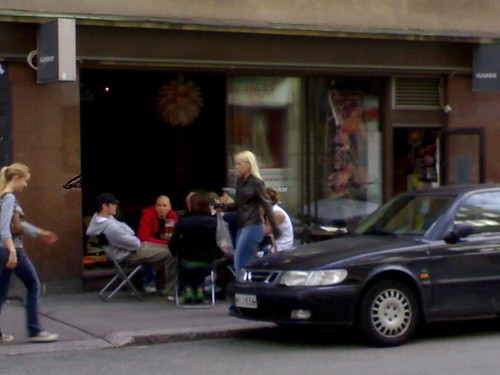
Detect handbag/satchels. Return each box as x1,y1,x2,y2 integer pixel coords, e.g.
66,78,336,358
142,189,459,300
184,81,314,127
0,192,26,237
217,212,236,256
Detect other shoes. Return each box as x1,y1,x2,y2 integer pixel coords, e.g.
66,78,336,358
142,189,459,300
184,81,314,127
29,330,58,341
143,283,157,293
166,296,182,301
0,333,14,342
183,288,205,303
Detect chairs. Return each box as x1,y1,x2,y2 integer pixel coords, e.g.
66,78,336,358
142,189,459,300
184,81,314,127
168,234,218,309
96,232,146,301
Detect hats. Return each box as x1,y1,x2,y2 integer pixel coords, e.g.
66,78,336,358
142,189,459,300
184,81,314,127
98,192,120,204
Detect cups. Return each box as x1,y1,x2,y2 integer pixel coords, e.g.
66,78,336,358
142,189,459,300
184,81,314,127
164,218,174,238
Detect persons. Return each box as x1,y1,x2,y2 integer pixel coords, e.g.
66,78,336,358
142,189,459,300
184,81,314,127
213,150,283,311
0,162,58,342
85,186,294,304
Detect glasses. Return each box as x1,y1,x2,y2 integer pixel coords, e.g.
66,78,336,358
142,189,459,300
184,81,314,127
333,143,342,147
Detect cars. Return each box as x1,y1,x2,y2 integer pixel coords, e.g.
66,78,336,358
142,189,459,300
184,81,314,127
228,184,499,347
299,181,383,242
